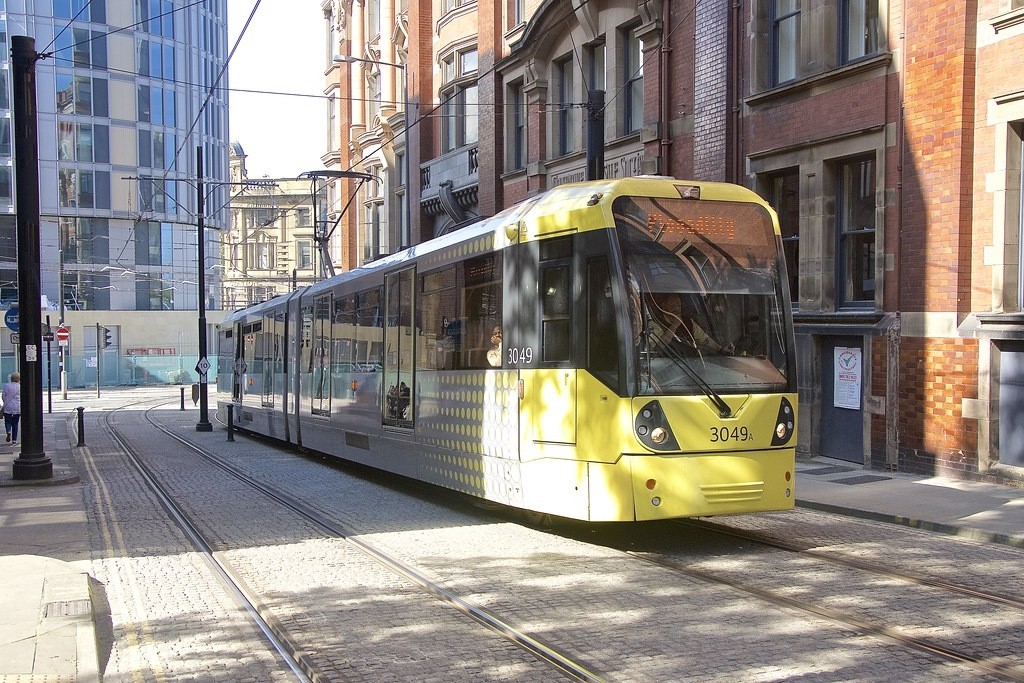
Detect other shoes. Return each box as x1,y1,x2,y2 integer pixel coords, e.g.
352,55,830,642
13,441,16,445
6,432,11,442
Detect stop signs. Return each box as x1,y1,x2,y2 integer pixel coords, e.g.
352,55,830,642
56,327,70,340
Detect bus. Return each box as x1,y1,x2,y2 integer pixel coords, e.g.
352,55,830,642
217,176,800,522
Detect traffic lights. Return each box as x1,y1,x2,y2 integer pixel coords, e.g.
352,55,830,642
102,327,112,350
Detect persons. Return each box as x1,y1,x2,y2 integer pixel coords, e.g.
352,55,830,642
2,372,21,444
649,292,735,356
487,326,502,366
386,381,410,418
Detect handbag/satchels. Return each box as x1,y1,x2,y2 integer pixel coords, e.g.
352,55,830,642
0,406,4,418
